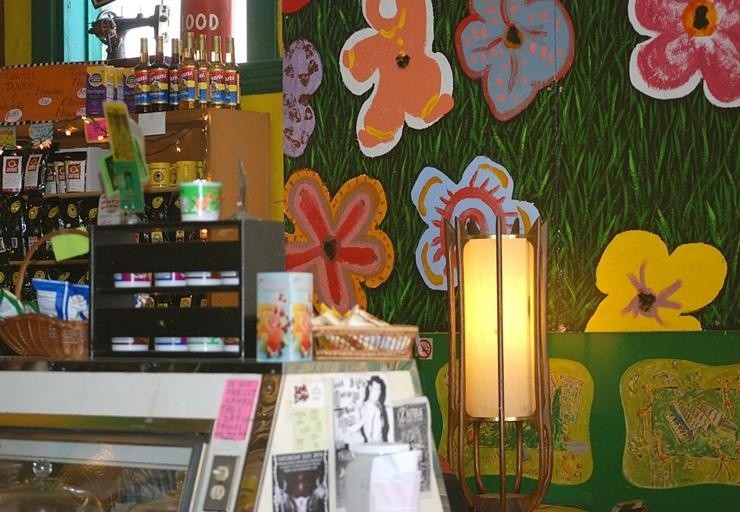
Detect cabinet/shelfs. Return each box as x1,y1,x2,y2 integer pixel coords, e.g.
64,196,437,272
125,109,270,220
0,140,90,359
91,219,284,361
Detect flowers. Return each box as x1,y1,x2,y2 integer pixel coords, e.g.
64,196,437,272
285,169,395,316
628,0,740,110
339,0,455,160
455,2,576,122
411,156,540,292
583,229,728,333
282,37,324,159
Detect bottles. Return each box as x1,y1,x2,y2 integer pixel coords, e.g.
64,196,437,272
209,35,227,108
150,36,170,112
133,38,150,113
169,39,179,110
179,32,199,110
227,39,241,110
199,33,209,109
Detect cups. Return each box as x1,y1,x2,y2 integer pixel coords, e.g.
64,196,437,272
146,161,204,189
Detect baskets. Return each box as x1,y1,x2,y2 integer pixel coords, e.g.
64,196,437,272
310,323,420,362
0,228,91,360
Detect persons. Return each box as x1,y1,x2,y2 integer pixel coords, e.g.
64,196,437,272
351,376,390,443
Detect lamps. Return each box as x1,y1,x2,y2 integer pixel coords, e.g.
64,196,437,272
441,215,553,510
